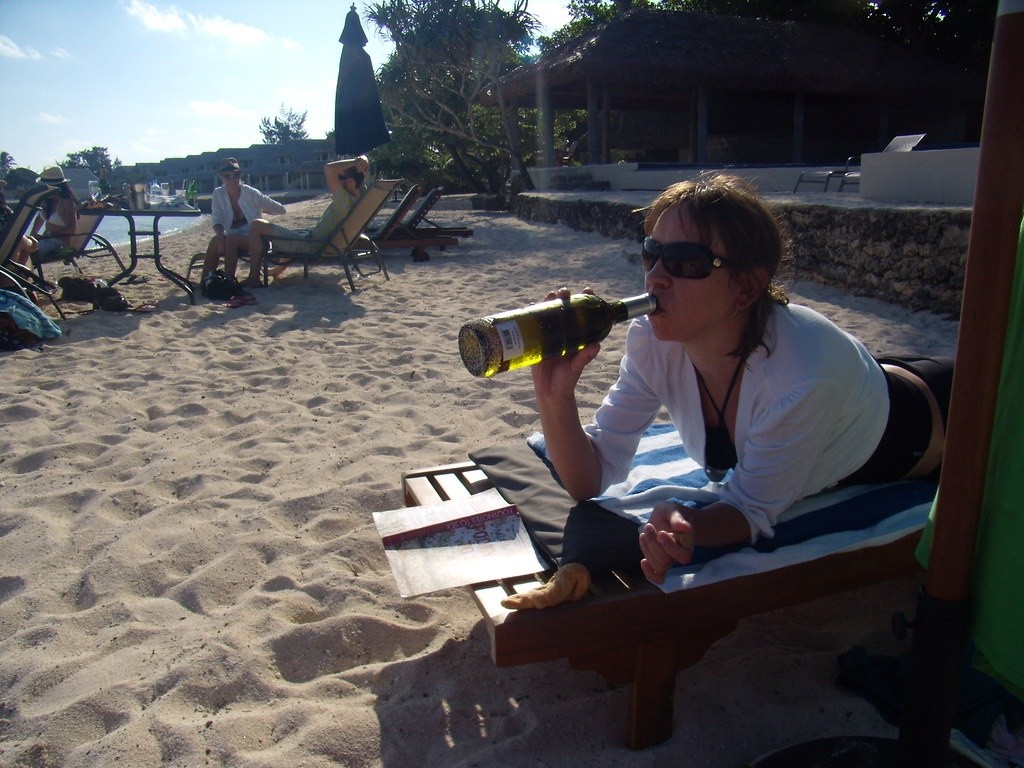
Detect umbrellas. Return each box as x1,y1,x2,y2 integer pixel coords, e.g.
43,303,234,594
333,2,392,159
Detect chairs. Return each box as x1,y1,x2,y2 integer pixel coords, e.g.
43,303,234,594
0,176,474,320
400,406,950,752
793,132,929,193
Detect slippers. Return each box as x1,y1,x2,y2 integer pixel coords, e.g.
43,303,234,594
222,294,257,307
126,303,160,312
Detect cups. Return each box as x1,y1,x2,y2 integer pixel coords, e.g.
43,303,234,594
91,187,102,202
89,181,100,198
175,190,186,197
161,182,170,196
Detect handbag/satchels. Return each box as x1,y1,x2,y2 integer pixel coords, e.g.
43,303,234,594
203,269,242,300
93,284,132,311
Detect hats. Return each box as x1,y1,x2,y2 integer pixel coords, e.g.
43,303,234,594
217,157,243,173
36,166,71,185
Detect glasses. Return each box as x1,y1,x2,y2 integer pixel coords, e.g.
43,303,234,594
641,237,754,279
222,174,240,179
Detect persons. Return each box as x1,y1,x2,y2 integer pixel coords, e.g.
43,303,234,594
195,156,286,290
528,172,955,585
238,155,369,289
17,165,81,268
0,180,14,247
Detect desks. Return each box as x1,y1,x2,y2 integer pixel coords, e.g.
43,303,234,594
78,208,201,305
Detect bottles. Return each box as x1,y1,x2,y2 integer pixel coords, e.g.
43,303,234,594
458,292,658,378
150,178,161,195
188,180,198,209
